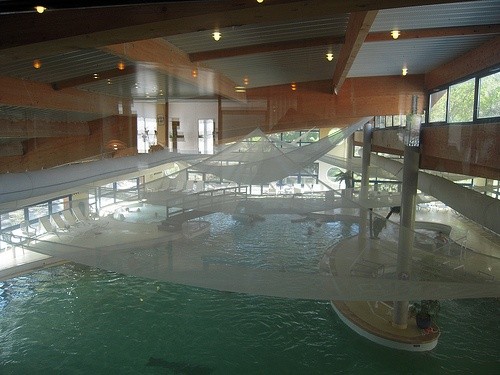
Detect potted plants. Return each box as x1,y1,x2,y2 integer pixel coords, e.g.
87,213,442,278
336,171,357,190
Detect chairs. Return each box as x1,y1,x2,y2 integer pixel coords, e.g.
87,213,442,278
20,206,108,247
170,181,204,196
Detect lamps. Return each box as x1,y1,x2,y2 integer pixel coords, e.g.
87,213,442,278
402,69,408,75
33,3,47,13
390,31,401,40
212,32,222,41
325,53,334,62
